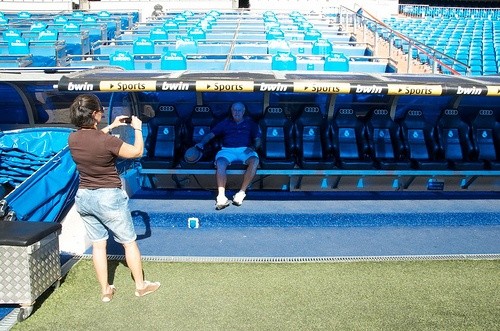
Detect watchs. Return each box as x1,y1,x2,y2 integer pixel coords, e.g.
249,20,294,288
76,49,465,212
108,125,112,132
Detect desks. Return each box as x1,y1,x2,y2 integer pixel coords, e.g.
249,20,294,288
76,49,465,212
0,14,398,74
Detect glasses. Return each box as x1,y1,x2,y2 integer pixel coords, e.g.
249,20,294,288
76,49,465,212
96,106,105,114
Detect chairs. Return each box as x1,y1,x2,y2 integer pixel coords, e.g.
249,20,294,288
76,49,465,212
0,11,349,71
124,106,500,176
354,6,500,78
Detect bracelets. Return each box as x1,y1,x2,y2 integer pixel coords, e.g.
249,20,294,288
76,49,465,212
133,127,141,132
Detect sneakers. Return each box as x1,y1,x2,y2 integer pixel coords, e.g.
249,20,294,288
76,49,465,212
135,280,160,296
216,196,230,209
101,285,116,302
233,193,243,205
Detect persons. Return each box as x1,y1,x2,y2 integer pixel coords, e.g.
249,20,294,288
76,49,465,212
69,94,160,303
215,102,262,211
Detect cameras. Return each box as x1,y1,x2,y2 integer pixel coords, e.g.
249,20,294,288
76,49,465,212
123,118,132,123
